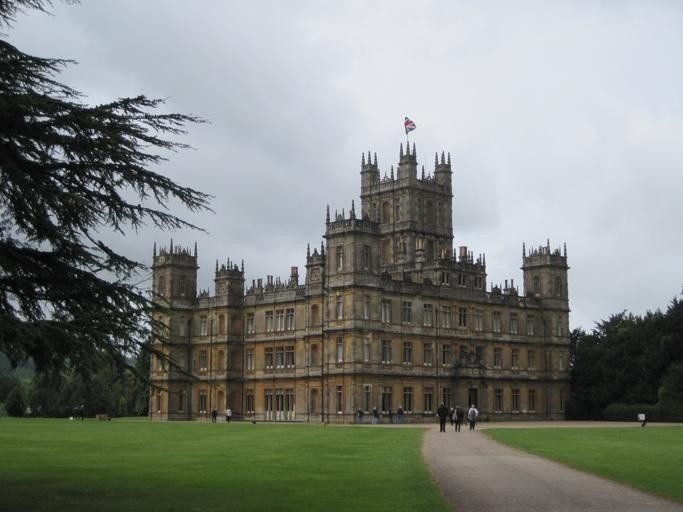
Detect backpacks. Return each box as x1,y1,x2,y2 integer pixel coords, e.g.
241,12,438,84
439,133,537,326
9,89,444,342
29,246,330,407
468,411,475,422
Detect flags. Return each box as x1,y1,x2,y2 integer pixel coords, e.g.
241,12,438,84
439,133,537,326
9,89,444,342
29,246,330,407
404,117,416,134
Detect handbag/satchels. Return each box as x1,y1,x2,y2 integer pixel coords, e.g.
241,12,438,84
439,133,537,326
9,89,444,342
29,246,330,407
452,414,457,421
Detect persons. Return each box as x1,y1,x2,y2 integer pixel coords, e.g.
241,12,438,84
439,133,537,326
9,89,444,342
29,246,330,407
358,408,362,423
437,402,479,432
211,408,217,422
78,404,85,421
36,405,42,418
71,405,80,420
397,405,404,422
371,405,378,424
226,407,232,423
17,403,24,417
637,410,649,427
25,404,32,415
